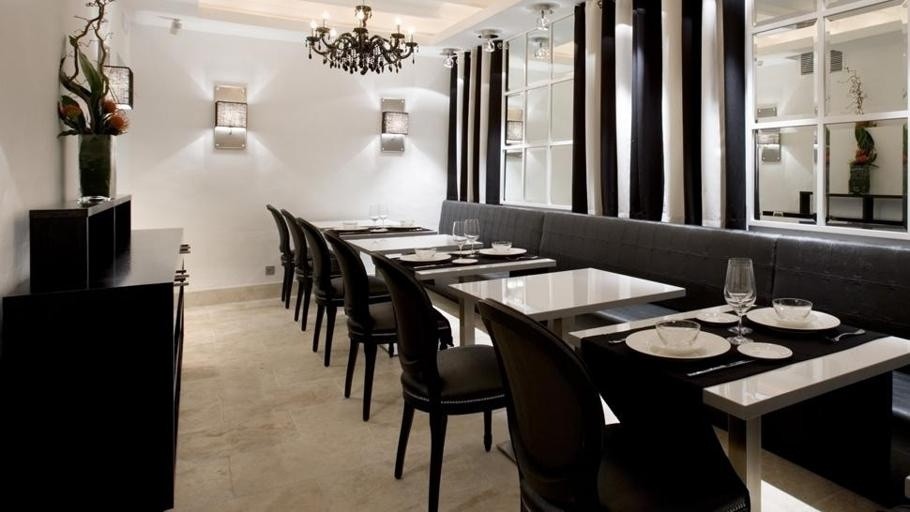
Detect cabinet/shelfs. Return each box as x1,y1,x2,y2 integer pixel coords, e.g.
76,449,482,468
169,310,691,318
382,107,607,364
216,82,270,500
0,194,188,512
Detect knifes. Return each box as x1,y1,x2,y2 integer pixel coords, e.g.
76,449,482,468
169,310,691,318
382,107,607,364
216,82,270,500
412,263,453,270
685,358,752,377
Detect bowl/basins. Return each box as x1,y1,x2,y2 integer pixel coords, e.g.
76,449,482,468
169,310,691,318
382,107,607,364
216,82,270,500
772,297,814,322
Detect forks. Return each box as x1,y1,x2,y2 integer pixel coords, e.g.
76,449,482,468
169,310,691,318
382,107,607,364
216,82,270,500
825,326,868,343
504,256,539,261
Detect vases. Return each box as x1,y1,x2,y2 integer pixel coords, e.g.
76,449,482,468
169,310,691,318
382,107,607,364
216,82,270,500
77,133,117,199
848,161,871,194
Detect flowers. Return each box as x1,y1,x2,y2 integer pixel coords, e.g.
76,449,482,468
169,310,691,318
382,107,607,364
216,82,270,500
56,0,129,138
839,69,879,165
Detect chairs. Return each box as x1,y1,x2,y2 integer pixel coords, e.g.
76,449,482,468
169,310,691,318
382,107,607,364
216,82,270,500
281,209,343,331
267,202,333,308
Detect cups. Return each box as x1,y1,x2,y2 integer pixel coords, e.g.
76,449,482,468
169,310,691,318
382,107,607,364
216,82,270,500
414,247,437,257
490,240,515,252
652,317,703,350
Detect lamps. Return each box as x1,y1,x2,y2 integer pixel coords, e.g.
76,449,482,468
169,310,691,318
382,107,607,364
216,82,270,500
305,0,420,77
381,97,408,152
531,4,560,31
100,66,134,110
531,37,549,59
214,83,247,151
506,108,526,152
476,29,502,52
439,47,461,68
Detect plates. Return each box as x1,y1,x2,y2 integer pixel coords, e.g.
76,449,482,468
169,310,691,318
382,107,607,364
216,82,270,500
399,252,451,262
625,324,732,362
479,247,528,257
696,311,742,327
383,223,422,231
737,341,794,360
746,306,842,333
451,258,480,266
332,225,369,231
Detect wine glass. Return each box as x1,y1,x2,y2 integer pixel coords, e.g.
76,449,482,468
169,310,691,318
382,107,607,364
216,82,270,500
724,257,757,335
368,205,388,233
450,218,481,264
